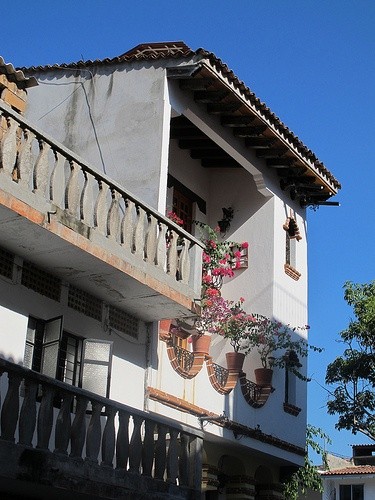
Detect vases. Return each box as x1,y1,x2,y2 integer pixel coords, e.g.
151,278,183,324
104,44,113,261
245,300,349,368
254,368,274,385
225,352,246,370
191,335,212,355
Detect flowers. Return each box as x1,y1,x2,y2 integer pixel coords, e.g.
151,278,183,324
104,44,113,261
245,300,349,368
191,288,233,336
222,296,281,358
244,313,324,382
164,207,248,288
